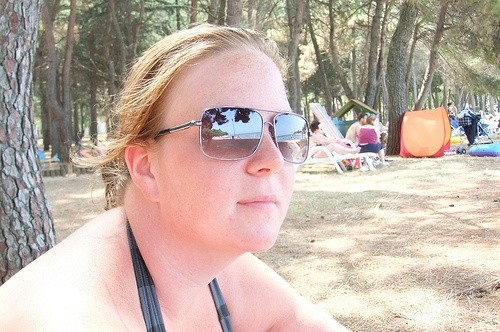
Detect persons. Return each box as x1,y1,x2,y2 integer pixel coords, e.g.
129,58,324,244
357,114,387,166
344,113,368,146
446,101,458,120
0,22,356,332
306,120,362,159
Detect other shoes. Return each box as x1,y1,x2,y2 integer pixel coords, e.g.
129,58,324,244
373,161,389,166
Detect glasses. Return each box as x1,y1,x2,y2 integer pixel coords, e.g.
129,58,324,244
154,105,313,164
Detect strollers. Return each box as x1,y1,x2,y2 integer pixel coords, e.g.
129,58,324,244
456,109,494,155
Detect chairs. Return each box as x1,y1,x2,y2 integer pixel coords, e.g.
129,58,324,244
296,101,378,174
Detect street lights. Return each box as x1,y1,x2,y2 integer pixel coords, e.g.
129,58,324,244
231,119,236,137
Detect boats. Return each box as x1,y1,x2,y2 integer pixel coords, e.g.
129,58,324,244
294,129,303,136
